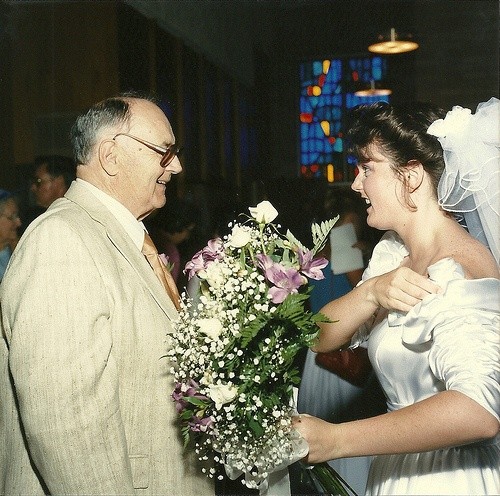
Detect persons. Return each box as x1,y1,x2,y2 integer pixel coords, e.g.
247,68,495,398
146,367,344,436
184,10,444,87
0,94,218,496
281,101,500,496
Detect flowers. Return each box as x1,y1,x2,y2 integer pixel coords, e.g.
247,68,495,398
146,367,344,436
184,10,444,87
158,200,359,496
159,253,175,273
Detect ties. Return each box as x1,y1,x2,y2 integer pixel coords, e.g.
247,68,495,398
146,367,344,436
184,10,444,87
142,229,180,315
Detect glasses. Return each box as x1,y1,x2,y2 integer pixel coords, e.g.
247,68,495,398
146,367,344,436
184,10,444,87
34,177,56,186
113,133,182,167
